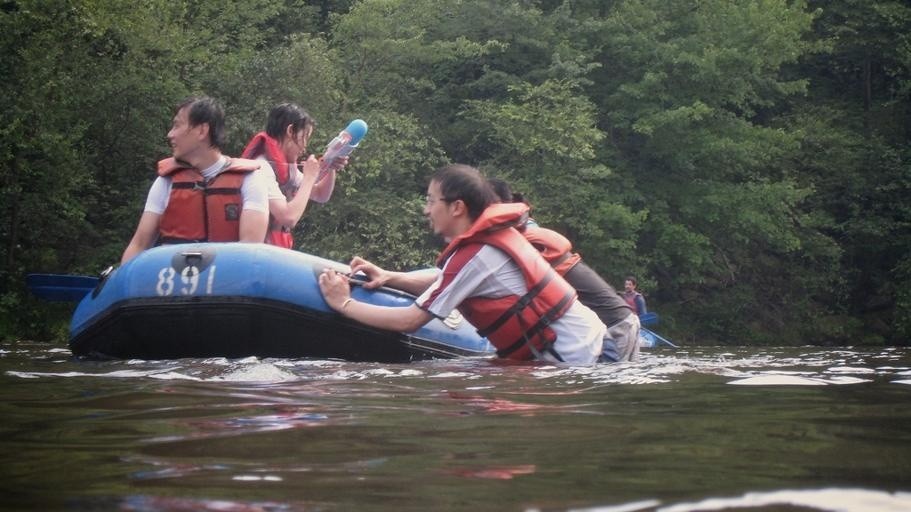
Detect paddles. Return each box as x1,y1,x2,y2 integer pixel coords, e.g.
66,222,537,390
638,313,658,326
27,273,99,302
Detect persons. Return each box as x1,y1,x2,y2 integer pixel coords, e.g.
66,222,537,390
621,274,648,315
241,102,371,248
319,164,607,369
119,95,269,265
488,177,642,363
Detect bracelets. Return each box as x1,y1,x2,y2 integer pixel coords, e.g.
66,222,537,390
340,298,353,307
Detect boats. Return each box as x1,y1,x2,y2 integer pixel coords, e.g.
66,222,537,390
65,241,659,367
